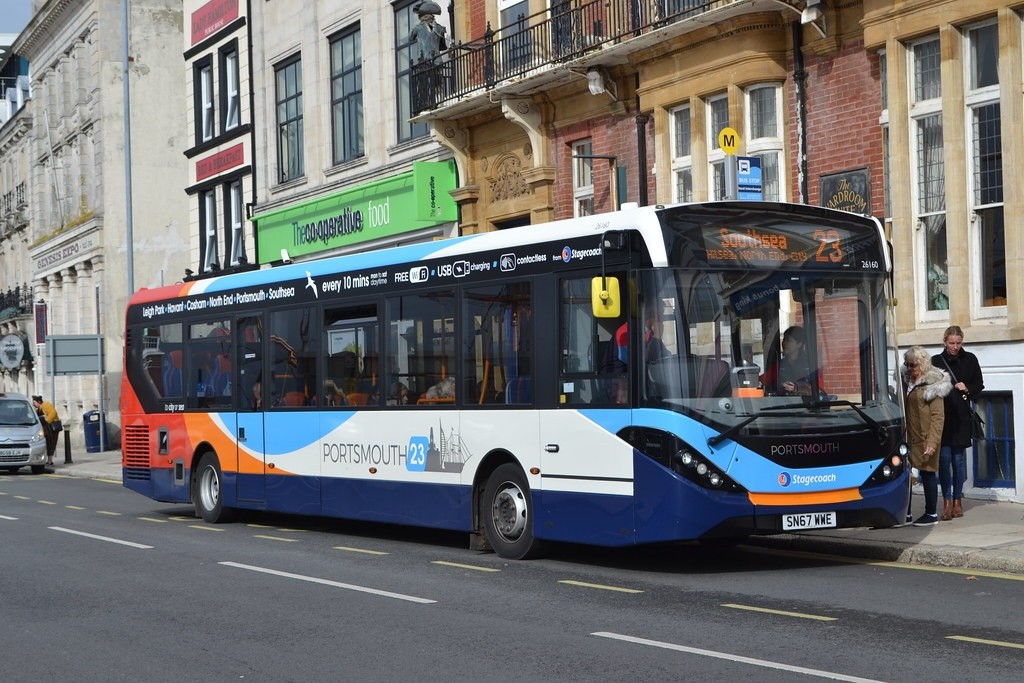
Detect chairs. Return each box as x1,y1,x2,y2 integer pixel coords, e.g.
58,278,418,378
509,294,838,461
170,350,426,407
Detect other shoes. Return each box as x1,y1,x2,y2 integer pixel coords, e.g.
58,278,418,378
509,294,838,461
44,462,54,468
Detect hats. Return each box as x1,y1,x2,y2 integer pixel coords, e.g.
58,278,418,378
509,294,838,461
784,326,805,343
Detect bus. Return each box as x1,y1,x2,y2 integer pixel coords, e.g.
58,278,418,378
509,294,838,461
119,199,912,563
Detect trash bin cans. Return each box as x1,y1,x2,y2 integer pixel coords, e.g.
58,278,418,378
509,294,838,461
83,410,107,453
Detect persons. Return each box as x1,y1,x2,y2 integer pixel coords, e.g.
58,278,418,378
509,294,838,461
602,292,673,401
409,13,443,108
427,358,456,403
886,346,953,528
32,395,59,467
931,326,984,520
759,325,824,396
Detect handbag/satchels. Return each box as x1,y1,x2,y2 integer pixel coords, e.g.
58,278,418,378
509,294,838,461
51,421,62,432
970,404,985,440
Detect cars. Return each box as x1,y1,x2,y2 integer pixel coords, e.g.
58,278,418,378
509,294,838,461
0,391,48,475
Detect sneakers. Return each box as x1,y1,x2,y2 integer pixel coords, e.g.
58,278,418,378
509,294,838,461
891,514,912,527
913,514,938,526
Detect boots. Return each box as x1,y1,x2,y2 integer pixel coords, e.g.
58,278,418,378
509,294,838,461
952,498,962,518
941,498,952,520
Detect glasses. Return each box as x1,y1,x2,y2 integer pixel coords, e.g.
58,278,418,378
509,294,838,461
904,362,917,367
